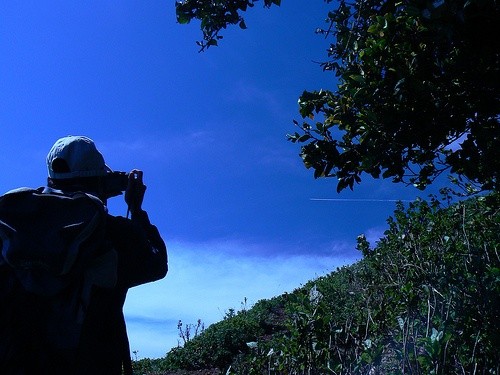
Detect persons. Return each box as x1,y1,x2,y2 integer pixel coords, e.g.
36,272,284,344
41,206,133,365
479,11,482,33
0,135,169,375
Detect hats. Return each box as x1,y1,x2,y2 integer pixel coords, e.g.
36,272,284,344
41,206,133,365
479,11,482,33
45,135,115,180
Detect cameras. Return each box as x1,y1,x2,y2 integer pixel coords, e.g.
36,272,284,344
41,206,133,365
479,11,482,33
105,171,138,191
